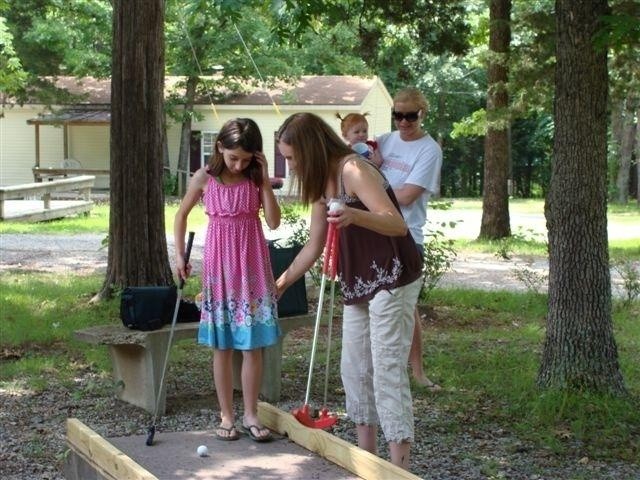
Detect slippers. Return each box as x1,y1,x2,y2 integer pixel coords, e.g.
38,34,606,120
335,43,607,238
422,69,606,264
241,425,273,440
216,425,238,441
416,382,442,392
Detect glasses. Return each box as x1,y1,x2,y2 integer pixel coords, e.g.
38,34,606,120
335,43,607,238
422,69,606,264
392,108,422,122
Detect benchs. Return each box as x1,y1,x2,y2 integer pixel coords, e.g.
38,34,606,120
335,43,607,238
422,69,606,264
74,310,331,414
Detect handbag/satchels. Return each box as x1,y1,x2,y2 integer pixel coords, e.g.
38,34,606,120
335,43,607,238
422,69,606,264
265,239,308,316
121,286,201,331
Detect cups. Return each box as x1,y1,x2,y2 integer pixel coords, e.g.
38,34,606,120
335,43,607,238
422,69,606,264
351,142,369,157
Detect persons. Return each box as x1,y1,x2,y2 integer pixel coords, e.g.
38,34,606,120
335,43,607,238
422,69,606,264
336,89,444,392
276,113,425,470
174,119,280,442
